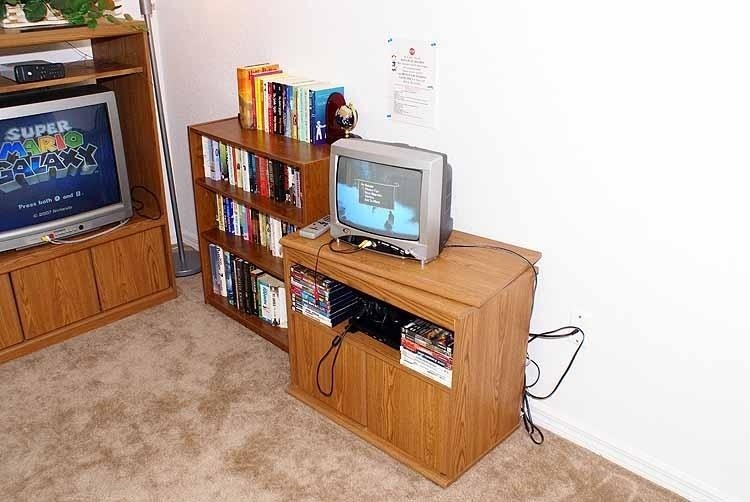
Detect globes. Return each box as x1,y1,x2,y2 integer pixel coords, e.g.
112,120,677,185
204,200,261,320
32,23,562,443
329,101,363,145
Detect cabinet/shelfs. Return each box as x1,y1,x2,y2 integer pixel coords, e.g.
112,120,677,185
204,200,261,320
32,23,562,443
1,18,177,365
186,116,330,353
277,221,543,491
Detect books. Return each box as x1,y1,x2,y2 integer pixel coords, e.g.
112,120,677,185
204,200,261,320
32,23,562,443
237,62,344,144
210,244,287,329
202,136,304,208
290,263,362,328
216,194,298,258
400,319,454,388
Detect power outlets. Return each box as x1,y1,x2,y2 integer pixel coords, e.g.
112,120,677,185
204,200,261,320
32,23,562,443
568,311,587,347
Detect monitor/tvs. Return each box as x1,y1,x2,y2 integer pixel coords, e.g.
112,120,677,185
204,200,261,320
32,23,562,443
0,84,134,253
328,138,453,265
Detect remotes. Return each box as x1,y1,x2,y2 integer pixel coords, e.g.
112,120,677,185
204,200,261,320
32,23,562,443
298,215,331,239
14,63,66,84
